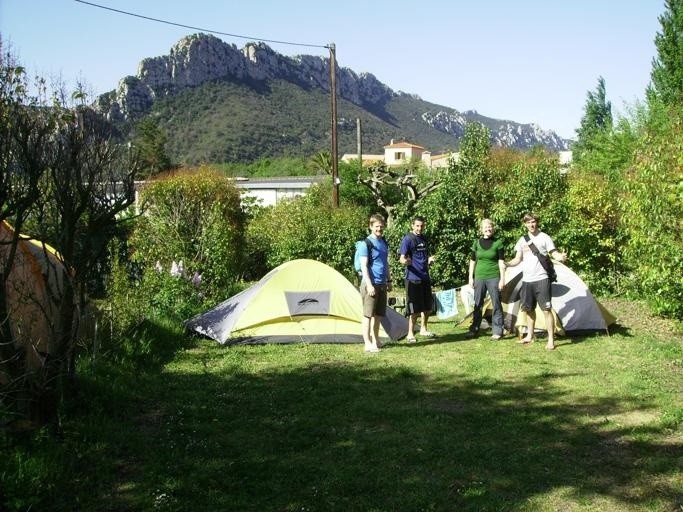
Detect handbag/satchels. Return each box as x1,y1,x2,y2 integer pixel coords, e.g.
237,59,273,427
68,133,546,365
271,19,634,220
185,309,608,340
537,254,557,279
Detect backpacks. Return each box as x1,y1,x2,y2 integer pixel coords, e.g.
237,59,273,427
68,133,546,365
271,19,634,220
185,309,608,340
354,240,372,276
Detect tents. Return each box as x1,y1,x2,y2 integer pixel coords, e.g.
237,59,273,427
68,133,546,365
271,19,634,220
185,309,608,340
182,258,422,348
1,217,84,376
460,256,616,339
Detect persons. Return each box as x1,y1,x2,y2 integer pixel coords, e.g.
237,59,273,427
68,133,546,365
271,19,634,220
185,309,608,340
400,216,436,340
503,213,567,352
468,217,504,342
355,214,388,354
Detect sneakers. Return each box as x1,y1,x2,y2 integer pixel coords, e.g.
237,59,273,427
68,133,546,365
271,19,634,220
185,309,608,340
517,339,535,343
489,335,499,341
363,341,383,354
406,334,416,343
467,330,482,340
420,332,436,339
545,344,554,350
